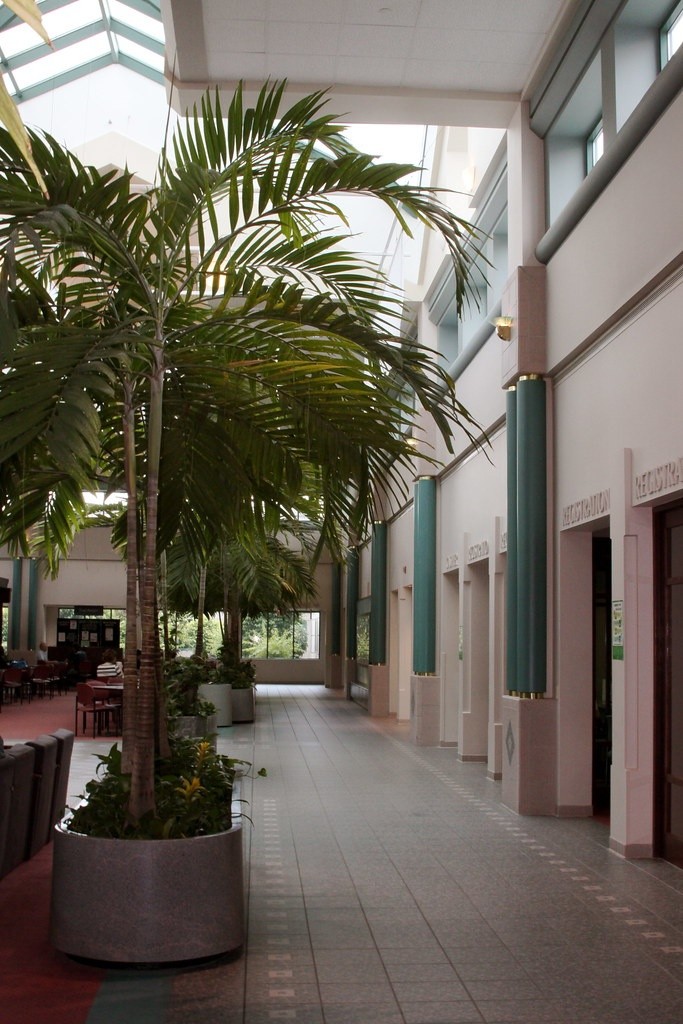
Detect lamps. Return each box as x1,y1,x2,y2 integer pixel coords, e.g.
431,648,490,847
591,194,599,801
489,316,512,340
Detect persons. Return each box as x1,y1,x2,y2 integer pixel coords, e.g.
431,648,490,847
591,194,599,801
64,640,103,689
97,648,209,679
37,643,48,665
0,646,8,669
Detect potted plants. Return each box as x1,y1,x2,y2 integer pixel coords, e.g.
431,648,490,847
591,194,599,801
159,500,218,755
174,517,321,727
0,74,496,964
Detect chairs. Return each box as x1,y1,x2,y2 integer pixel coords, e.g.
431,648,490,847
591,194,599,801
1,656,125,739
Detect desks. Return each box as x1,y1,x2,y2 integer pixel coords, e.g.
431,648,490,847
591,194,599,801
92,685,123,692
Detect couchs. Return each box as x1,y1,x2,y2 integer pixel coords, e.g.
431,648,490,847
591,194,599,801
0,727,76,879
